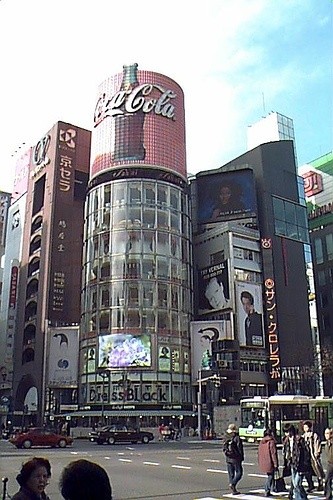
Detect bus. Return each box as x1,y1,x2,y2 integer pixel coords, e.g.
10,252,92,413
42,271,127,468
238,394,333,446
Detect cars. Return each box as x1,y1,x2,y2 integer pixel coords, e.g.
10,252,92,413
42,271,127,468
89,424,156,444
9,428,73,449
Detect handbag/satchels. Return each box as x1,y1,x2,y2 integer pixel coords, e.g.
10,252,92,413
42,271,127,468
273,467,286,493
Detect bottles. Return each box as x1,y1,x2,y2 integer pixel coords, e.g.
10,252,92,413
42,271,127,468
274,470,279,480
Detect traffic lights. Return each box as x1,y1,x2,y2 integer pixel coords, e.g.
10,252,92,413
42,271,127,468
208,377,220,387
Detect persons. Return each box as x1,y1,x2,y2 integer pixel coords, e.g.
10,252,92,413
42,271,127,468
212,181,245,217
158,422,179,441
204,277,229,308
282,424,308,500
323,427,333,500
258,429,278,496
300,421,325,492
188,426,212,440
223,423,244,494
11,457,52,500
61,459,113,500
241,291,263,346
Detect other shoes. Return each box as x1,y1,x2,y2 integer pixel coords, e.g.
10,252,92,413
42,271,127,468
306,486,315,492
232,490,240,495
229,483,235,493
317,486,324,491
265,490,273,496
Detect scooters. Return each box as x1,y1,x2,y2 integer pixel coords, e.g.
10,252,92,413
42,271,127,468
163,430,176,442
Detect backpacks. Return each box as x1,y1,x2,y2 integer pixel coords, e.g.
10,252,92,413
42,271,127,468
222,435,241,465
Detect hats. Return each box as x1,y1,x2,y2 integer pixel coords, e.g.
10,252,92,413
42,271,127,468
226,424,239,433
